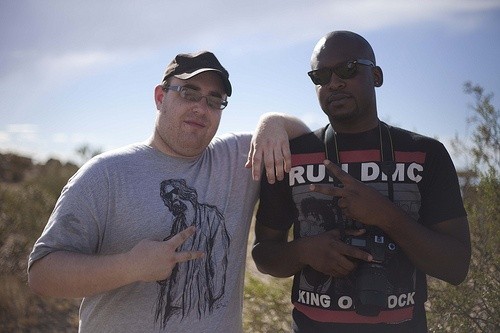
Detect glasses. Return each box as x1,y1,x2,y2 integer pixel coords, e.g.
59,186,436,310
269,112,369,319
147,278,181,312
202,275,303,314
308,58,376,85
162,86,228,110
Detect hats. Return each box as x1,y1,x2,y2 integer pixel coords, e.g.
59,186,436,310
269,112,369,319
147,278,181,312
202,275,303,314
163,51,233,97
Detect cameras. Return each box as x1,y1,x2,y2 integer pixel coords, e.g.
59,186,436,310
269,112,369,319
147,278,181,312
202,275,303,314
343,229,399,265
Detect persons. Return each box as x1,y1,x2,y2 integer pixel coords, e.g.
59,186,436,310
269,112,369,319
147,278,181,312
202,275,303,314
251,29,472,333
27,51,312,333
